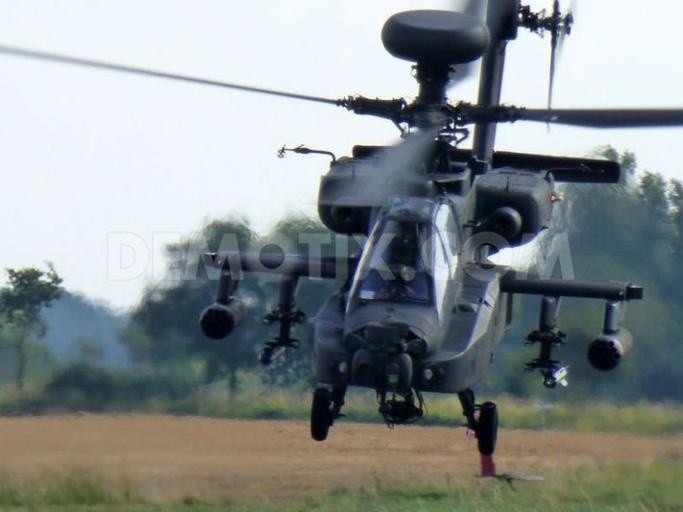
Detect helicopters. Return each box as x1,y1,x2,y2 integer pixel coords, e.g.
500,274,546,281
0,0,683,458
